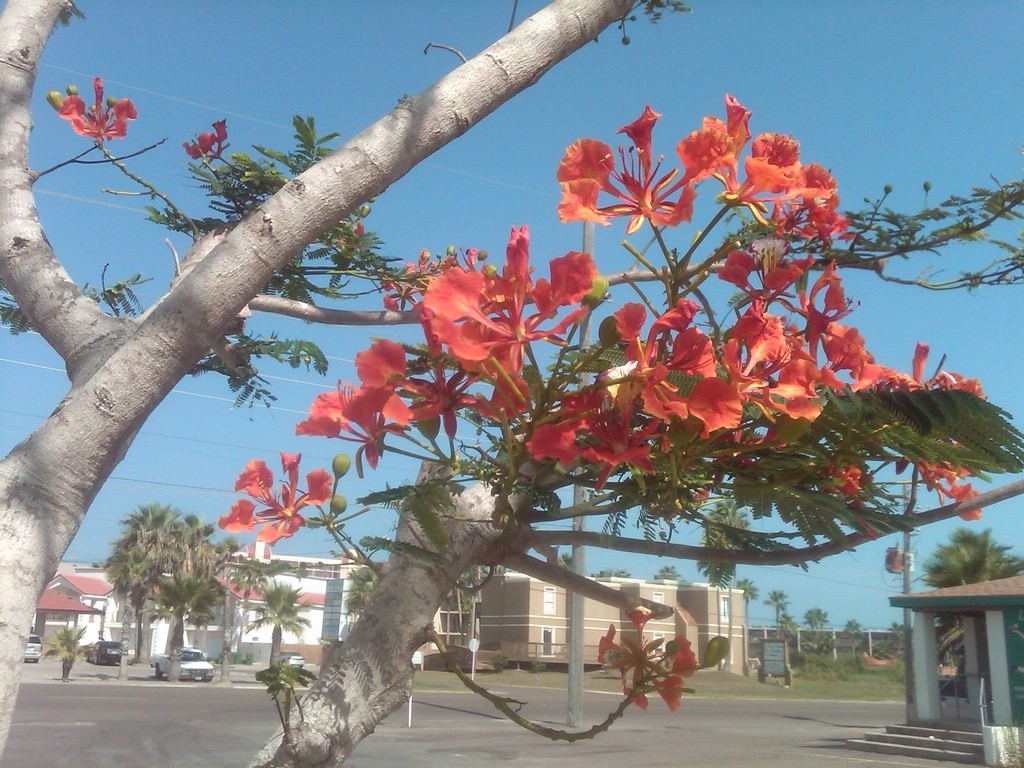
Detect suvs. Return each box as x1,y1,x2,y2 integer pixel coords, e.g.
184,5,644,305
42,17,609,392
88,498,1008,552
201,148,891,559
25,632,43,663
86,641,124,665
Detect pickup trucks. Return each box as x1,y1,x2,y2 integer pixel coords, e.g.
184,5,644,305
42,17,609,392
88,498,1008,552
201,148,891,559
150,647,215,682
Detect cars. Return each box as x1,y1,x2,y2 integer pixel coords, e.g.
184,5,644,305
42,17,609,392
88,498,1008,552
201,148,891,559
279,651,305,669
